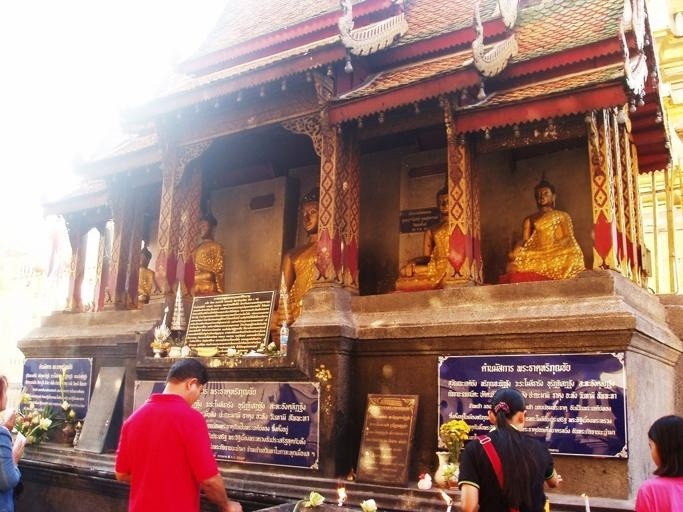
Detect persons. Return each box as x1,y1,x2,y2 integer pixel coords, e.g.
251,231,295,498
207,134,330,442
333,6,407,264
455,383,563,512
137,245,161,302
496,179,586,282
632,414,683,512
191,211,227,296
259,186,325,343
0,375,26,511
389,187,451,291
110,357,246,512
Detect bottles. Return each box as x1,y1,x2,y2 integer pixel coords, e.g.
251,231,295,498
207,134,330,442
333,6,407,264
168,347,181,357
279,322,289,356
72,426,82,449
434,451,450,488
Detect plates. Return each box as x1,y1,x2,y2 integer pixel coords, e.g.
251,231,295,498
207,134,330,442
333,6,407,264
242,353,269,358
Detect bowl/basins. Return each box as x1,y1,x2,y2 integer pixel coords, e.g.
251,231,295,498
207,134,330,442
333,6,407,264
189,346,219,357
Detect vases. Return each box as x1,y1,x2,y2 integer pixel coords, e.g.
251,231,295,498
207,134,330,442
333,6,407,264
434,452,461,489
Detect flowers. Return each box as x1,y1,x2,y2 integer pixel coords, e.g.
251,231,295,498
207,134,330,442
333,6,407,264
11,392,76,464
438,420,471,464
